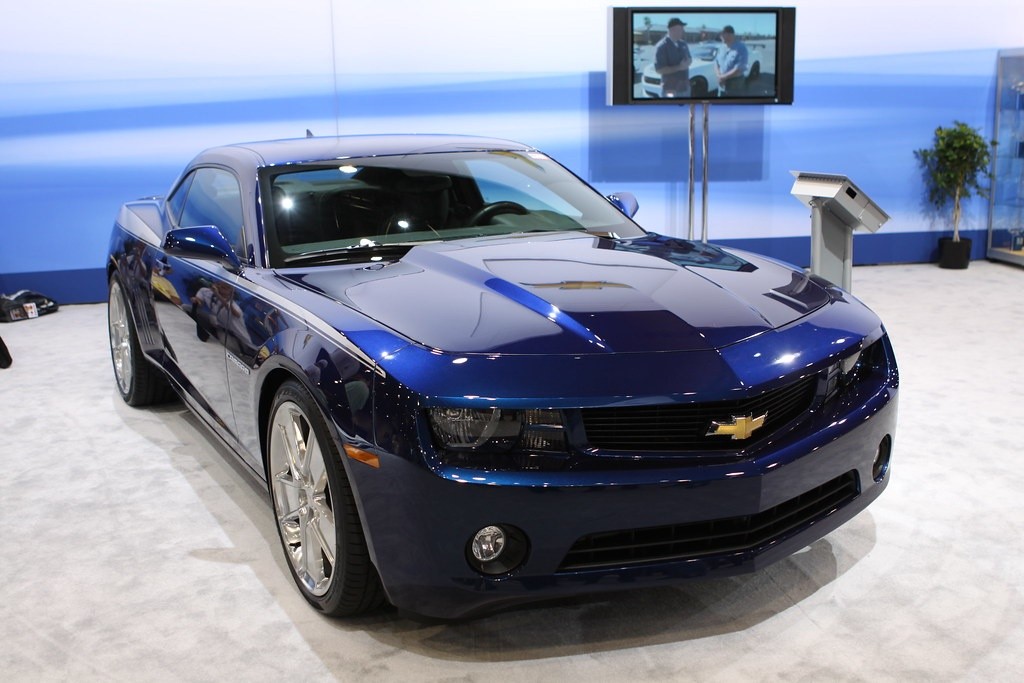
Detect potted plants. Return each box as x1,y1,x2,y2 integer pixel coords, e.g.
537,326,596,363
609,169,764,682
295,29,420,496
913,119,1000,268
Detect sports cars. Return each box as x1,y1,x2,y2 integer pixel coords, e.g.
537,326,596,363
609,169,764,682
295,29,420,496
642,40,766,97
104,129,901,621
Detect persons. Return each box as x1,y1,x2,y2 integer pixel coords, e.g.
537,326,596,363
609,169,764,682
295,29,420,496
653,18,692,98
714,26,748,97
304,360,328,387
344,374,370,413
182,281,252,353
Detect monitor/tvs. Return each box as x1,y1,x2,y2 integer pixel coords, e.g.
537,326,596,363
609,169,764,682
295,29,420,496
605,6,796,105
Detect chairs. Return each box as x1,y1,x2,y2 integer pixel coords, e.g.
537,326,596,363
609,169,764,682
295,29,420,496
399,173,459,230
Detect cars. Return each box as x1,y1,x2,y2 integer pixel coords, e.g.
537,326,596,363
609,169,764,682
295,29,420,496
705,60,707,62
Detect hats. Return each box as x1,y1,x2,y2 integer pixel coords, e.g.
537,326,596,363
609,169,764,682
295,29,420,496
668,18,687,28
722,26,734,33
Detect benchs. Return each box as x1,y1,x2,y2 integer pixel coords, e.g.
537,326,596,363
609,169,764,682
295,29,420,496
316,183,385,240
